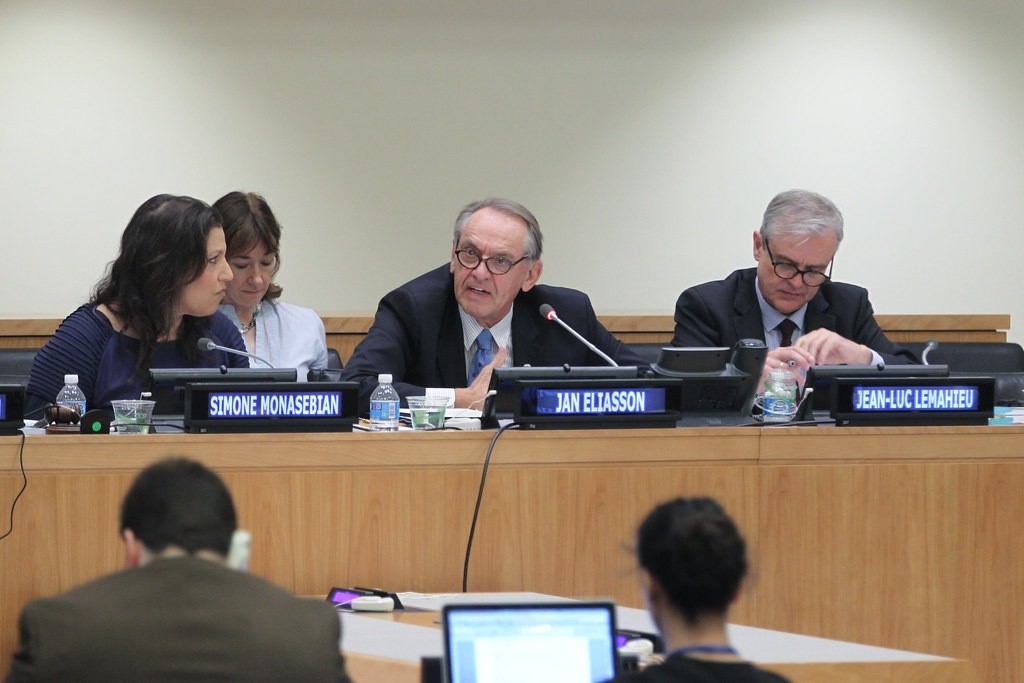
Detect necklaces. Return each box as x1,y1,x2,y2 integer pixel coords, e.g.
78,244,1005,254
669,645,736,656
240,301,262,333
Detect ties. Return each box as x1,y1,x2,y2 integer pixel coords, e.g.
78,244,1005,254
468,328,493,387
777,318,797,347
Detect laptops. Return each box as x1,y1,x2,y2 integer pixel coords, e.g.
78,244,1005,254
442,600,621,683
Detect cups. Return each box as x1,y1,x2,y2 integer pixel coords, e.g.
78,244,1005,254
406,396,451,431
752,363,804,415
110,399,156,434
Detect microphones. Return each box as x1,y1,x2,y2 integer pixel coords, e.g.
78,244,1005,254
538,303,618,367
198,338,277,368
922,341,938,365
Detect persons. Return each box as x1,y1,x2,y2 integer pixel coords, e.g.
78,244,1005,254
5,456,352,683
671,190,907,396
213,191,328,382
338,198,650,410
25,194,250,421
604,496,794,683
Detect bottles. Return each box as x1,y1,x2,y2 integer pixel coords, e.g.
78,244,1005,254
763,374,797,422
55,373,86,417
369,374,400,432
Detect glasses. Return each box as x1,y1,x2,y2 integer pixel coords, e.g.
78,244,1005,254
765,237,834,286
455,237,530,275
